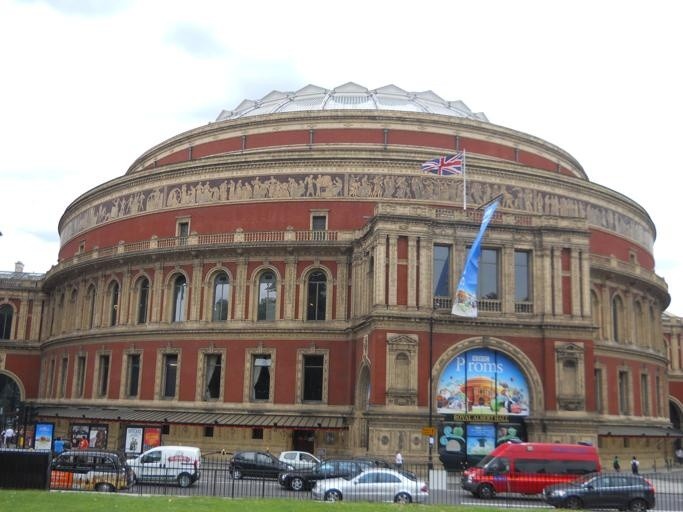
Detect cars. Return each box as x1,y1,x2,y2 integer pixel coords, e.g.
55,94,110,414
537,473,655,511
228,449,296,480
278,451,321,471
277,455,429,505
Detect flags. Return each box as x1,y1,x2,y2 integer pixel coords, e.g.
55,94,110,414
422,149,464,178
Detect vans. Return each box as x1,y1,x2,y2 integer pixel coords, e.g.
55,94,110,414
124,446,201,488
45,451,136,492
460,436,601,498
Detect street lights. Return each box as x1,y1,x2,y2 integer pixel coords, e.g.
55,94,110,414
426,304,441,462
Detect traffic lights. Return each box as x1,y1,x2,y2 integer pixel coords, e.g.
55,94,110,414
11,404,41,425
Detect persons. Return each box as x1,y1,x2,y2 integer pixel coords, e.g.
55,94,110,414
71,434,79,448
80,436,88,449
1,425,35,448
629,456,640,474
54,436,65,454
613,454,622,472
395,450,403,468
675,446,683,463
